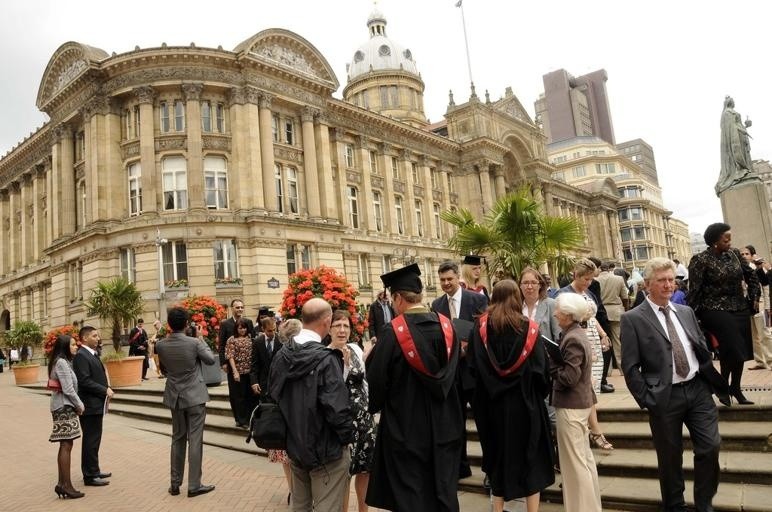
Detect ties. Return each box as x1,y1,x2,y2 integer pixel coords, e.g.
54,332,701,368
128,331,139,344
448,298,457,320
659,307,690,378
266,338,272,351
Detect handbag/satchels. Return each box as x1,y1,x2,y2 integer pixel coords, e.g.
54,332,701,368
747,295,759,315
47,379,61,390
246,401,286,450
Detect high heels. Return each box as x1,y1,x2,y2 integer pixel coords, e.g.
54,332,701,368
729,387,754,404
60,488,84,498
719,399,731,406
55,485,80,498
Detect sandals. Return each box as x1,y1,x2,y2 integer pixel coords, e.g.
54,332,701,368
590,434,614,450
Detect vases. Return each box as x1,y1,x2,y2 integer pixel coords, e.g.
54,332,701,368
201,354,222,387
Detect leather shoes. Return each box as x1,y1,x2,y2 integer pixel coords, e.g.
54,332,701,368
84,477,110,486
748,365,766,370
84,472,111,480
601,384,614,393
187,485,215,496
169,487,179,495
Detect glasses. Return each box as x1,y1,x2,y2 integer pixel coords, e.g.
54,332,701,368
331,324,349,329
232,306,244,309
521,282,540,286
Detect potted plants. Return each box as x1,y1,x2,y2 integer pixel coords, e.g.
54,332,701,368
0,320,43,387
82,275,148,387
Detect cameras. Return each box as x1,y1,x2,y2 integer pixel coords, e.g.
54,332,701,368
754,256,765,265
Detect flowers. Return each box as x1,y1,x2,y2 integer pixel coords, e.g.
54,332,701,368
156,295,228,353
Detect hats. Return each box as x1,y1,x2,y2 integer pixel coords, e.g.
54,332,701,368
380,263,421,298
464,255,486,265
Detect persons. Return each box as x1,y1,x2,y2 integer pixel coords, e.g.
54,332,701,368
468,278,555,512
621,258,727,510
433,254,500,347
519,267,562,341
714,96,758,192
156,304,216,496
586,257,649,393
368,287,397,345
73,325,115,485
670,222,770,406
364,263,472,512
541,274,557,298
553,258,615,450
329,308,377,512
545,292,604,512
218,299,283,430
145,321,163,378
128,319,149,382
47,334,85,499
266,298,356,511
0,343,30,374
265,319,302,504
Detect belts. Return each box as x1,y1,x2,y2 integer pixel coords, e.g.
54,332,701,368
673,375,697,386
